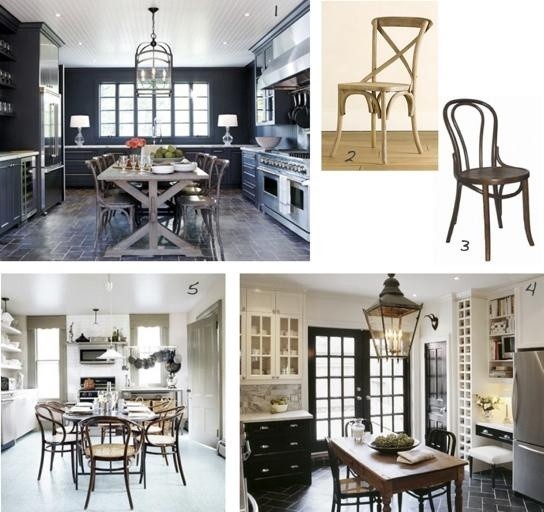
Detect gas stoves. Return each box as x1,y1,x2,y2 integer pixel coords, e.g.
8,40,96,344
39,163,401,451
255,149,310,180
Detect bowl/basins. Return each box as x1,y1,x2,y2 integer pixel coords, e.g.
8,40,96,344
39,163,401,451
255,136,281,150
151,156,197,174
271,404,287,412
0,325,22,369
250,315,298,376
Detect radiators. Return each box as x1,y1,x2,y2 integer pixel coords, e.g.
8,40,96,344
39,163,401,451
330,431,468,512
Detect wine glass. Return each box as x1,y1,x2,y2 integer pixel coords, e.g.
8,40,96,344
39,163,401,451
0,38,17,116
118,153,152,176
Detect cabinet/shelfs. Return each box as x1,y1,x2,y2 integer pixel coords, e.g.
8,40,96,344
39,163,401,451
65,148,242,187
16,22,65,94
1,323,22,370
0,158,21,235
515,276,544,348
0,50,16,118
16,389,37,439
240,288,306,385
244,410,314,491
241,151,258,205
457,298,487,461
249,32,292,126
487,294,515,383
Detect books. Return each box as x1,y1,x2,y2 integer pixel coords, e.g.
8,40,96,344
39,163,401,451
489,295,514,378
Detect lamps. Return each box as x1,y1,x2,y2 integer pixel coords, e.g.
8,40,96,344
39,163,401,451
217,114,238,145
361,273,424,362
135,8,174,98
1,298,14,321
90,309,100,328
97,273,124,359
70,115,90,146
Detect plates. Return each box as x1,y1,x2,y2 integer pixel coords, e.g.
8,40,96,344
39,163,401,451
368,436,420,454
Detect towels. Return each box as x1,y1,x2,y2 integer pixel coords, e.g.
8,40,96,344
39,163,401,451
397,449,434,464
279,176,291,214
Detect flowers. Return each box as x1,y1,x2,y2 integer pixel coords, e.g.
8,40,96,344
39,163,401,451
127,138,146,149
474,393,503,410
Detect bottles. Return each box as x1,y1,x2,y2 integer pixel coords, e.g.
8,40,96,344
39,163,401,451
111,329,124,341
92,381,128,416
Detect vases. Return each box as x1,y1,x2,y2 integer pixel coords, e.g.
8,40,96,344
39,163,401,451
482,410,493,423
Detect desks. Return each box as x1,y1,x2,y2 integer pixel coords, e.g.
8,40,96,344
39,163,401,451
476,422,513,445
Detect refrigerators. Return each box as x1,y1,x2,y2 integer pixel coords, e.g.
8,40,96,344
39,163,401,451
511,349,544,505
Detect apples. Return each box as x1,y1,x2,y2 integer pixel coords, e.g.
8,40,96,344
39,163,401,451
155,145,184,158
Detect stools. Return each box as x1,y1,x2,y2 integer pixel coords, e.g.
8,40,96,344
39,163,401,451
469,446,512,488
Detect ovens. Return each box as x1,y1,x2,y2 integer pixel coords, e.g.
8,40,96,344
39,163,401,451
240,421,255,512
255,163,310,245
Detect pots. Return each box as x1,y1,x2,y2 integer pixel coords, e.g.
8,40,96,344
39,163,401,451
288,91,310,129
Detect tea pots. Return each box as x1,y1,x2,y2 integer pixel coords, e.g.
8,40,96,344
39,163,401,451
349,419,367,443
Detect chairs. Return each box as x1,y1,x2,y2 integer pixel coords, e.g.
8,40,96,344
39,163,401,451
34,395,187,510
398,429,456,512
86,153,230,254
345,421,374,479
330,16,433,165
326,438,382,512
443,98,533,261
